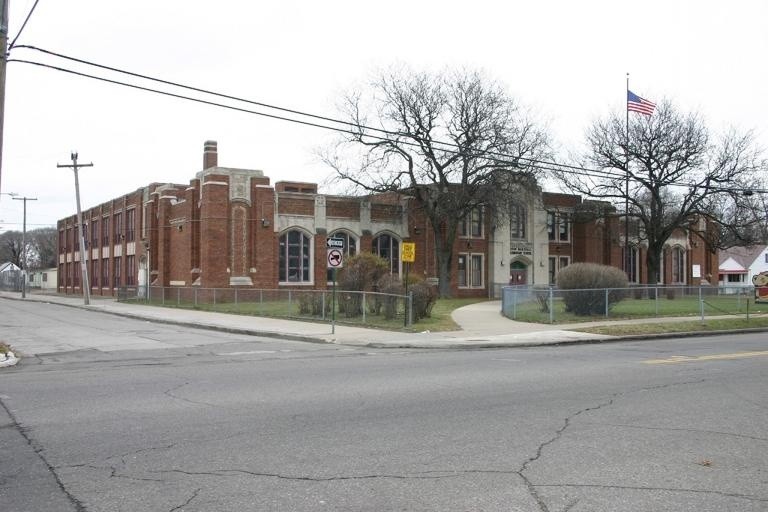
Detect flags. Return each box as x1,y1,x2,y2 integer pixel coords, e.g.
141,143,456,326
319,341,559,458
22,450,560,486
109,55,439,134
627,89,657,116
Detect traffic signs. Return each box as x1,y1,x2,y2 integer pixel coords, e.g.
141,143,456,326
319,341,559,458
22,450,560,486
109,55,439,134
327,238,344,249
